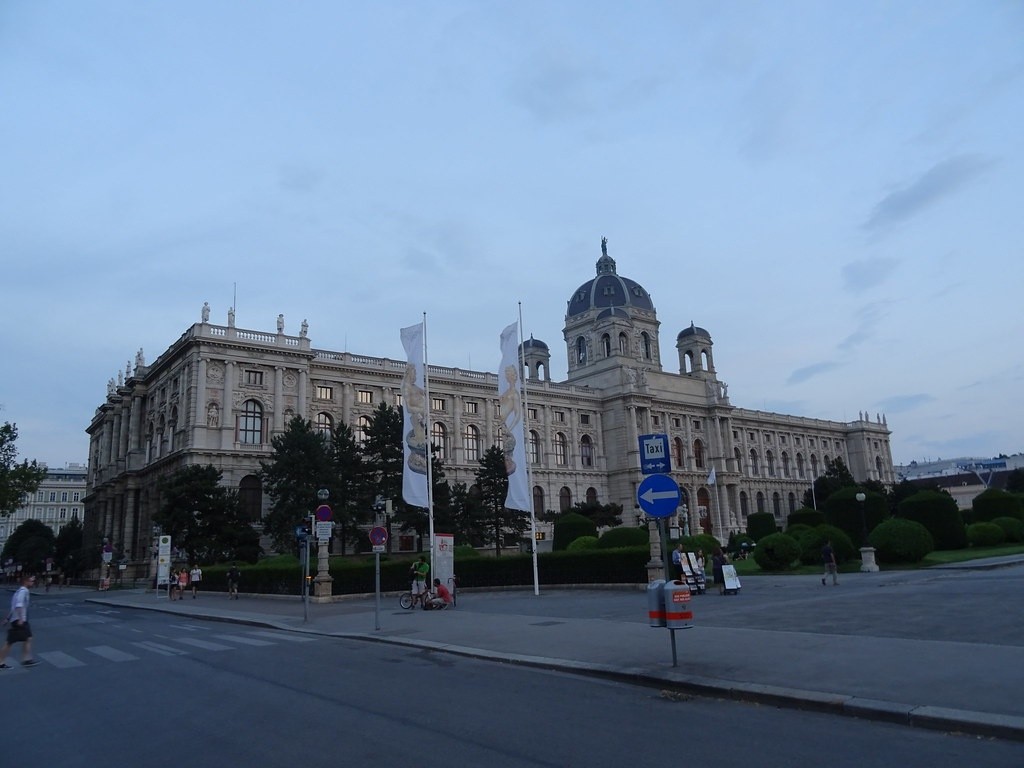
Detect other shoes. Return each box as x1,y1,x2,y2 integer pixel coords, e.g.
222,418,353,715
0,664,14,670
22,661,39,667
822,578,826,586
236,595,238,599
420,604,423,608
434,605,442,610
228,596,232,600
444,605,448,610
833,583,839,586
193,595,196,599
179,597,183,600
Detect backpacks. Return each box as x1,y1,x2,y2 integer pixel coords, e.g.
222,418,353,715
170,574,176,583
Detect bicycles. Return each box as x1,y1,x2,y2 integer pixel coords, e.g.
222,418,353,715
399,583,439,610
448,574,460,606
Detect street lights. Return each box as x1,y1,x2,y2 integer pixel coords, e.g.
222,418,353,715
855,492,872,547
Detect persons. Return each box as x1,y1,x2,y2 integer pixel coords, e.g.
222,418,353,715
169,564,203,601
820,539,839,586
671,544,730,593
285,411,293,433
600,235,607,256
208,405,218,426
277,314,308,337
410,555,452,609
6,570,72,593
858,410,886,425
227,563,241,600
724,382,728,397
107,348,143,394
742,540,756,552
202,302,235,326
0,574,43,669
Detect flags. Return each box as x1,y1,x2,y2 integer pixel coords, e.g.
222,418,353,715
706,468,715,485
498,321,532,513
398,323,427,506
